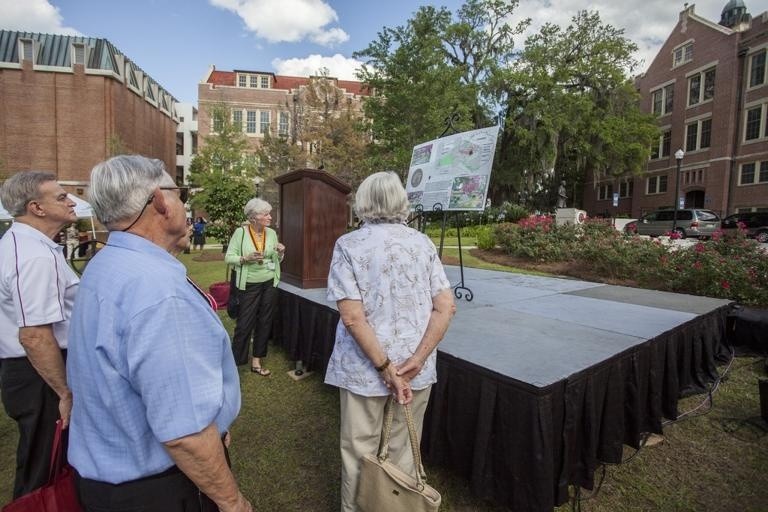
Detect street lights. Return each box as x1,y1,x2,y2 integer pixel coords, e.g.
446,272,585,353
670,148,686,238
253,178,259,198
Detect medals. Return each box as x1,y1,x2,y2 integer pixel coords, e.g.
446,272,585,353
258,259,264,265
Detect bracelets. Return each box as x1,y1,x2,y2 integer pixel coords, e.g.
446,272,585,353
374,356,391,372
243,256,247,263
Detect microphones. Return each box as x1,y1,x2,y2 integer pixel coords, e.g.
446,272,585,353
317,165,324,169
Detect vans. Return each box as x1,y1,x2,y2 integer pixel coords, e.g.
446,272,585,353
623,209,721,241
719,211,768,243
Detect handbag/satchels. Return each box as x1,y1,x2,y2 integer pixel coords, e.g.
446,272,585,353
226,268,241,318
355,453,442,512
0,465,82,511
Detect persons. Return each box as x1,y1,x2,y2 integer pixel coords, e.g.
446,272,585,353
0,168,80,500
66,152,257,512
223,197,287,376
183,218,195,254
322,169,456,511
193,217,207,251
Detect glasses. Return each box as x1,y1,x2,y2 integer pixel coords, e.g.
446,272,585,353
147,185,190,204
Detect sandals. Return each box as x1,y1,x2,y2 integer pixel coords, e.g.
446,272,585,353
250,367,271,376
